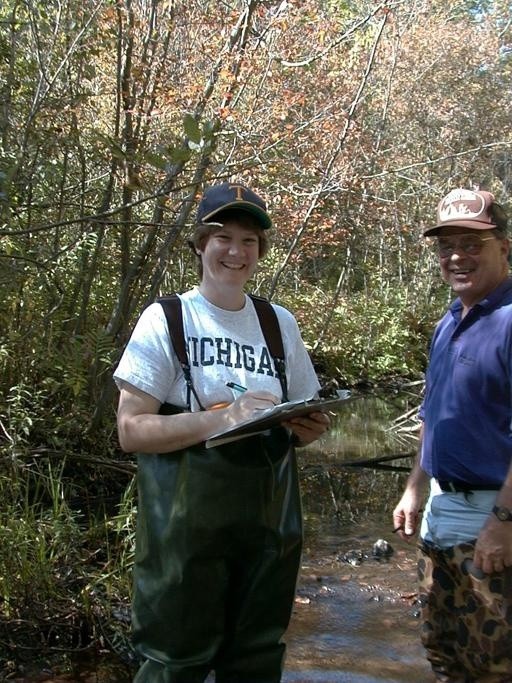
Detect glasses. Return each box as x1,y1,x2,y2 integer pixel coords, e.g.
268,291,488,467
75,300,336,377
436,234,498,255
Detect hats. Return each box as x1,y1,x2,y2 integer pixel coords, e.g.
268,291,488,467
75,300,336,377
424,190,506,237
197,183,272,230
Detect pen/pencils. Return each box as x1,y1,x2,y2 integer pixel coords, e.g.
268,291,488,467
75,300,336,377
392,509,422,533
226,382,277,406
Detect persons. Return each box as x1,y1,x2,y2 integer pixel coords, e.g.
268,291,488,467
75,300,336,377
116,181,332,683
391,188,512,683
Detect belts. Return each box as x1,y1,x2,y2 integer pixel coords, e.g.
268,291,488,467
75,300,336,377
440,480,503,492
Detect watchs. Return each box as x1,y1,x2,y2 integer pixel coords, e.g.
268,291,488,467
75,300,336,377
492,505,512,521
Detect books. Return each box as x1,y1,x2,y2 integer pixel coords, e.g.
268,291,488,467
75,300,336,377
204,388,352,450
